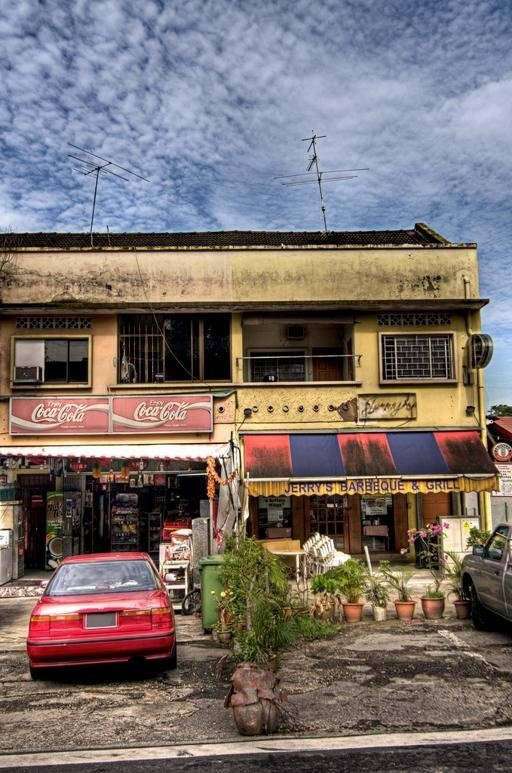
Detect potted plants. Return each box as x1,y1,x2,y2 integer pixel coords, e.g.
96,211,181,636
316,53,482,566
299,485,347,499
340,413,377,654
311,558,415,623
425,547,471,619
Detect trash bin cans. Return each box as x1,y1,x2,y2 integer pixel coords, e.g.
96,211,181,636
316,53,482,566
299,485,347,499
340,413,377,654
196,553,237,635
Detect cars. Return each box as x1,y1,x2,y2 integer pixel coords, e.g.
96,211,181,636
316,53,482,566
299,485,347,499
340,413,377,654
458,523,512,635
25,551,180,677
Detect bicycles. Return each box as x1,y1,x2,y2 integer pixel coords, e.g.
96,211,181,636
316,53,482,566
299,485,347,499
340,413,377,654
179,565,202,614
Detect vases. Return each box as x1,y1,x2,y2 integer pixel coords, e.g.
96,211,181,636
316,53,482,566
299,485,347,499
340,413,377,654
421,594,444,620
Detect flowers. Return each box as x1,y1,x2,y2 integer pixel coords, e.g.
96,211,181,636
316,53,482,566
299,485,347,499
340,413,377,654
400,519,449,597
